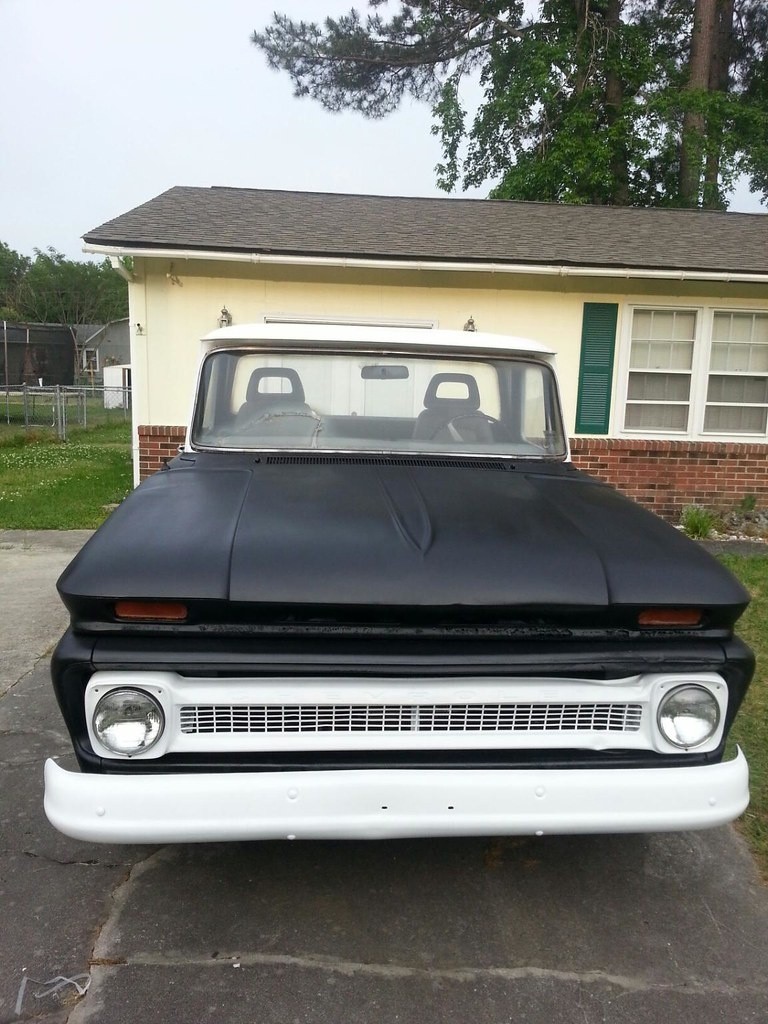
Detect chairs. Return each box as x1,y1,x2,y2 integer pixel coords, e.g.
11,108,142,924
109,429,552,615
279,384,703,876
233,367,311,429
413,373,492,443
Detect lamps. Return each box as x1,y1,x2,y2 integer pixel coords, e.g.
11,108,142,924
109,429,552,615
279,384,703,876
218,305,232,328
464,316,478,333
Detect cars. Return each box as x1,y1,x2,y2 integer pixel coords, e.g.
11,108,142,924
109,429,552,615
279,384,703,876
41,317,753,842
